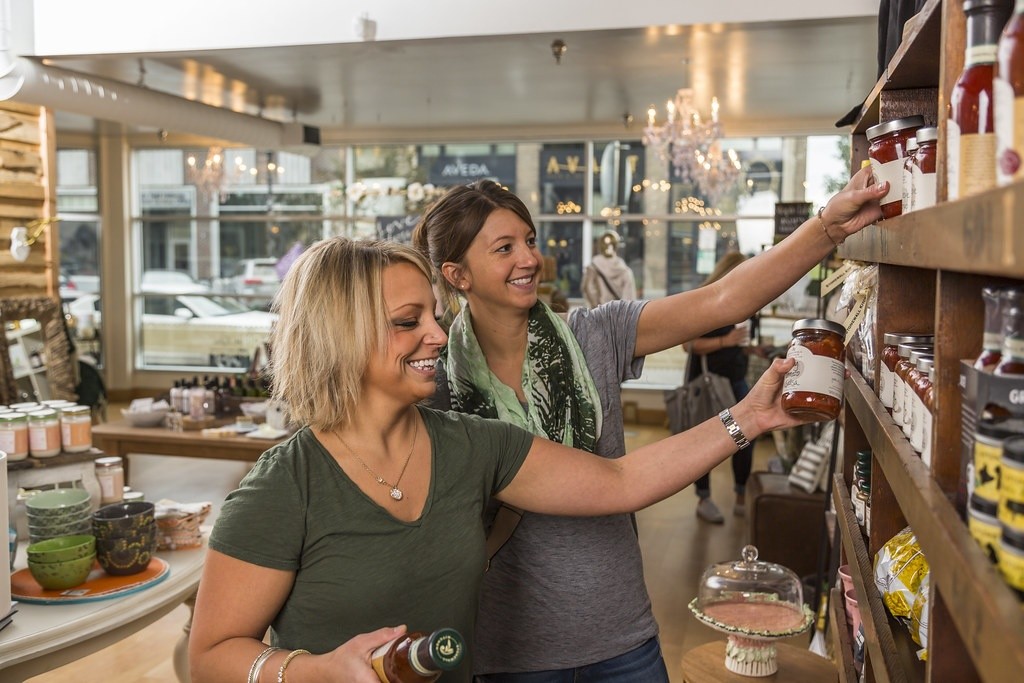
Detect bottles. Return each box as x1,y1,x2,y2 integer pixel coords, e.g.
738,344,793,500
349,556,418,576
949,1,1024,200
370,628,466,683
171,375,272,417
968,285,1024,594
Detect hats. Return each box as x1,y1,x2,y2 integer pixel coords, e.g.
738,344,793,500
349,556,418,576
602,230,620,245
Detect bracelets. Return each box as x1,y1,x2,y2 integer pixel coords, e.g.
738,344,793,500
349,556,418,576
719,409,749,450
719,337,723,348
247,647,283,683
817,206,836,243
277,648,312,683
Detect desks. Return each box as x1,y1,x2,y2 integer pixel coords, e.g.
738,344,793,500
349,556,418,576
92,419,288,487
681,639,839,683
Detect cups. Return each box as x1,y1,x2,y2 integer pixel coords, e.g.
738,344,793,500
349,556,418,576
0,450,157,621
77,313,95,340
735,319,751,347
838,565,863,641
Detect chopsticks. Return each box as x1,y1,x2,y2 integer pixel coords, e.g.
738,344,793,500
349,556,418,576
0,609,18,632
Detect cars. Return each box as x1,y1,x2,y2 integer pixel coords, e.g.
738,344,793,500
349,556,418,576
53,269,282,371
211,258,282,311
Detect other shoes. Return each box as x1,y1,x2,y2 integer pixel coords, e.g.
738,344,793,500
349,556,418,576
733,504,746,515
697,498,725,524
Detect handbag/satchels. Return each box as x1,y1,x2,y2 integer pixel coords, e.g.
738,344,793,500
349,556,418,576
663,339,736,435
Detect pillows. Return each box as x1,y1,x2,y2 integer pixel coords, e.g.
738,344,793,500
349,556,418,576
747,354,771,390
790,420,844,493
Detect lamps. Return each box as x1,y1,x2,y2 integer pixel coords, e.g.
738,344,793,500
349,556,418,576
642,33,742,206
185,146,227,197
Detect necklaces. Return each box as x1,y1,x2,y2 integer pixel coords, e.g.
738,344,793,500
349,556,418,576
332,405,417,500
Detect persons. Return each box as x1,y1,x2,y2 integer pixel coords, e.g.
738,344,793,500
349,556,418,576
681,249,753,524
185,235,816,683
410,168,891,683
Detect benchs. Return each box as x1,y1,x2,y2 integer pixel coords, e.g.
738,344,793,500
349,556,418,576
746,346,827,576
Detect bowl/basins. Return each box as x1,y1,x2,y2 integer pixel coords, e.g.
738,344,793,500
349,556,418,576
118,409,168,426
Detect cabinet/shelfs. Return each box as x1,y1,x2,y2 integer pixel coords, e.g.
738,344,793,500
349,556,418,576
829,1,1024,683
0,447,213,683
